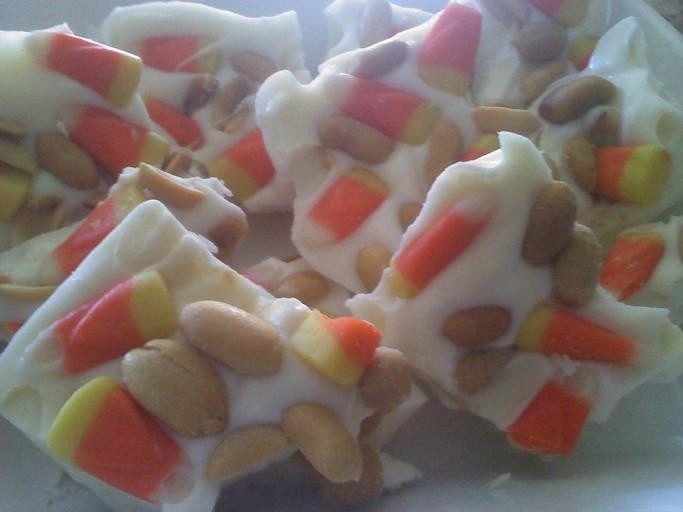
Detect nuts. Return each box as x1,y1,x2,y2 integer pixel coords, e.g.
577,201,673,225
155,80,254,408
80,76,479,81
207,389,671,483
0,0,618,485
